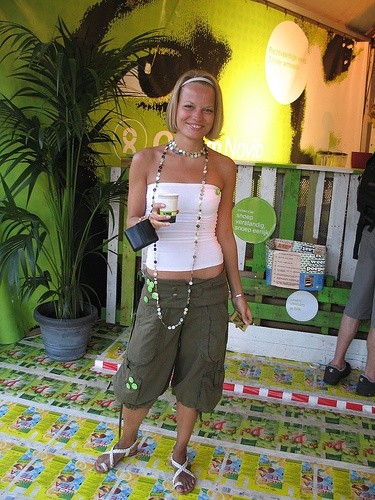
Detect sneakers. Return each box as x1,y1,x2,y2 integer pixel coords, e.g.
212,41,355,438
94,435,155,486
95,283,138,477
356,374,375,397
323,360,351,385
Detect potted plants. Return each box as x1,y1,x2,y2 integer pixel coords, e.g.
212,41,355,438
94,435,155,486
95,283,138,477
0,1,167,361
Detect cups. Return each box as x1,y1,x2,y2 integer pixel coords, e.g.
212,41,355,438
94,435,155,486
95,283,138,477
352,151,372,168
124,219,159,252
158,194,179,223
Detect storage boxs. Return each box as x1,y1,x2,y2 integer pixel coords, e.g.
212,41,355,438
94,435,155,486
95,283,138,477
265,239,326,292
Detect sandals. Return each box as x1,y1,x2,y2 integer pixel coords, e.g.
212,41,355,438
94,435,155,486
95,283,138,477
170,451,196,495
94,437,138,473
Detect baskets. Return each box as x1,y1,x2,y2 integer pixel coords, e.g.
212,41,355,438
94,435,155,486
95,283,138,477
265,238,327,292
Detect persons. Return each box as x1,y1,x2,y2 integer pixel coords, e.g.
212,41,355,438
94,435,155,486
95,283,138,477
94,69,252,495
323,152,375,396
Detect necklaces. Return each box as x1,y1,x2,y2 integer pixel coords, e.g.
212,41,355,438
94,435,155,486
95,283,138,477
150,137,208,330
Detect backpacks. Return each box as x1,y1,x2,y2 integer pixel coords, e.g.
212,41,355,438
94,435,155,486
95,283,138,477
352,152,375,225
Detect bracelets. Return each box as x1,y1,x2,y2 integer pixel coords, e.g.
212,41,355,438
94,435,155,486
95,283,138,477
231,293,245,298
138,214,151,223
